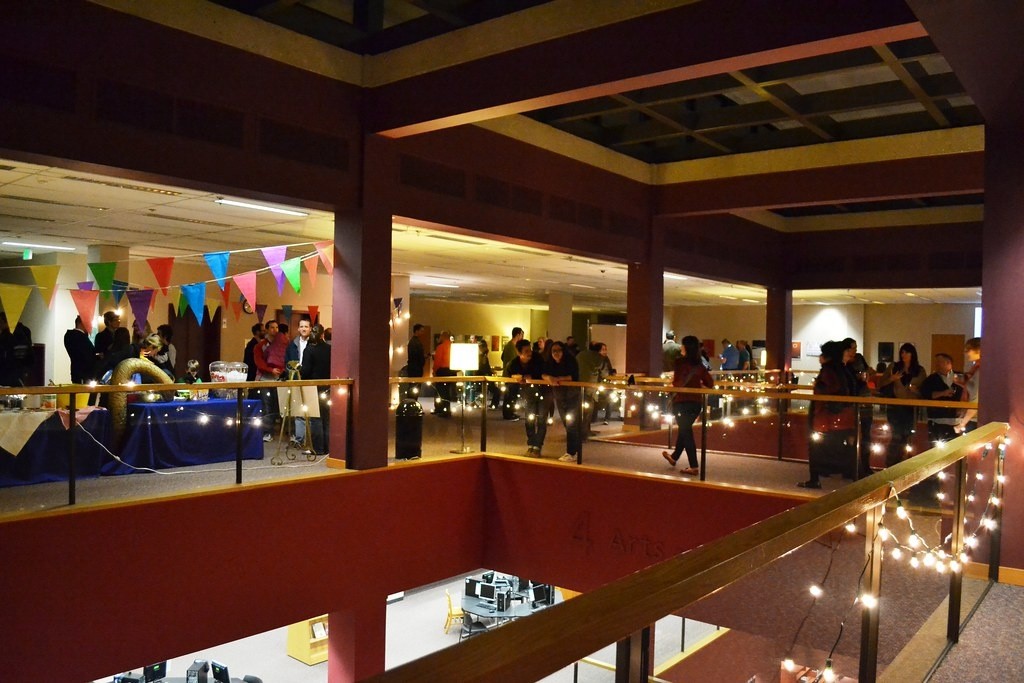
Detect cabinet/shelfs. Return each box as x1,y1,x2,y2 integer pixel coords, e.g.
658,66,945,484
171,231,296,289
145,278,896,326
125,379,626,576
287,614,328,665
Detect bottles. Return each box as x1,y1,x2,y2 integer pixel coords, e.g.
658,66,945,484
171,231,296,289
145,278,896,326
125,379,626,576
39,383,57,411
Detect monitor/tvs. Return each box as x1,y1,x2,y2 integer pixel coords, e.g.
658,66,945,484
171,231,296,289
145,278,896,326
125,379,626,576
531,584,546,608
479,582,497,608
482,570,494,583
143,661,166,683
211,659,230,683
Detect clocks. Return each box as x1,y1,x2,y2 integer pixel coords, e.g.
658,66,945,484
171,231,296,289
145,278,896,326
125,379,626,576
243,300,253,314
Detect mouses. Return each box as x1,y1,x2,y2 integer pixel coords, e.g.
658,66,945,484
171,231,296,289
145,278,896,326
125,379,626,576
489,610,494,613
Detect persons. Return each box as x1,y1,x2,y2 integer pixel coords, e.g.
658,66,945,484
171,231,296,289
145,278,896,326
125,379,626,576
432,327,618,463
947,337,980,441
405,323,429,403
918,353,969,448
63,310,206,407
660,335,714,475
241,315,332,457
661,329,762,372
797,337,875,490
876,342,928,467
0,311,33,386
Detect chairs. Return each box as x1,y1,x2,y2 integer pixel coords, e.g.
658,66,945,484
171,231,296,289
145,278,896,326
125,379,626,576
444,589,467,633
459,608,487,644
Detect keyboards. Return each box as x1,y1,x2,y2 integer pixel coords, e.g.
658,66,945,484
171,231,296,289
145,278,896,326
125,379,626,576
476,601,497,610
532,605,546,613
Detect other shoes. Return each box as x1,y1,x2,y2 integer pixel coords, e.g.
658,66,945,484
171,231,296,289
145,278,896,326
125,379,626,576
604,421,608,425
504,413,519,421
797,481,821,488
525,445,541,458
302,450,329,455
430,410,451,416
662,451,676,466
585,431,600,436
681,468,698,475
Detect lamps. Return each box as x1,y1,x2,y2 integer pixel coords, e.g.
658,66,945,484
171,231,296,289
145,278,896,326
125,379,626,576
449,342,479,455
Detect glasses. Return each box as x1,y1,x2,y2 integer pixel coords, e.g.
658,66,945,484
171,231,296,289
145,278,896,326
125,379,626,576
552,349,562,353
965,347,976,351
112,318,121,322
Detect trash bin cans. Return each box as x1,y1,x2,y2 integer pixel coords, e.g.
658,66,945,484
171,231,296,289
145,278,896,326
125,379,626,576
394,398,426,460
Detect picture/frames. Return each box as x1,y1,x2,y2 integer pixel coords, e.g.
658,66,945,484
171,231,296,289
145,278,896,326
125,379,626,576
878,342,893,362
502,336,509,351
433,334,440,351
791,340,802,360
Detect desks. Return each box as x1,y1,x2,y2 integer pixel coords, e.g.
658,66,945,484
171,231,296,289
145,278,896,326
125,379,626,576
461,586,563,627
101,398,264,474
107,678,248,683
0,406,108,487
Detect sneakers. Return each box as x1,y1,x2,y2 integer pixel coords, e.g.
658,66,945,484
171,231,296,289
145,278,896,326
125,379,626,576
263,434,273,442
559,453,577,462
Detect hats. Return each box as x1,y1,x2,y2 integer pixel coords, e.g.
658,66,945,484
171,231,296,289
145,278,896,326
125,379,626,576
147,334,163,351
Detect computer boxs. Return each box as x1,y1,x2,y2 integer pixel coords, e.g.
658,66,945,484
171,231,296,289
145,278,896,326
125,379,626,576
497,590,511,612
546,584,555,605
512,575,528,592
121,672,145,683
465,576,486,598
186,659,208,683
